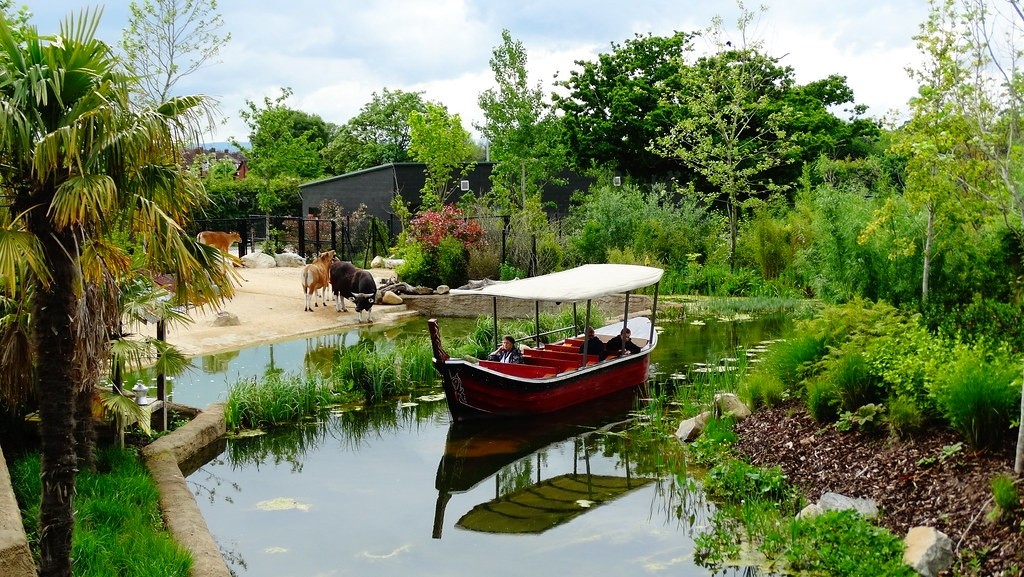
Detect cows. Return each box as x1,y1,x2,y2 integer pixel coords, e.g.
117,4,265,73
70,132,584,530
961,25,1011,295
232,252,310,268
197,231,242,253
298,250,405,323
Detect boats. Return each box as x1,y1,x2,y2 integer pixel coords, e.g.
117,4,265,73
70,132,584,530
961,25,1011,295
433,383,662,542
419,263,665,414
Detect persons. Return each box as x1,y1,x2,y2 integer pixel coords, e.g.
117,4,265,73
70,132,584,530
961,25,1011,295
580,325,606,362
606,327,640,355
487,335,525,364
